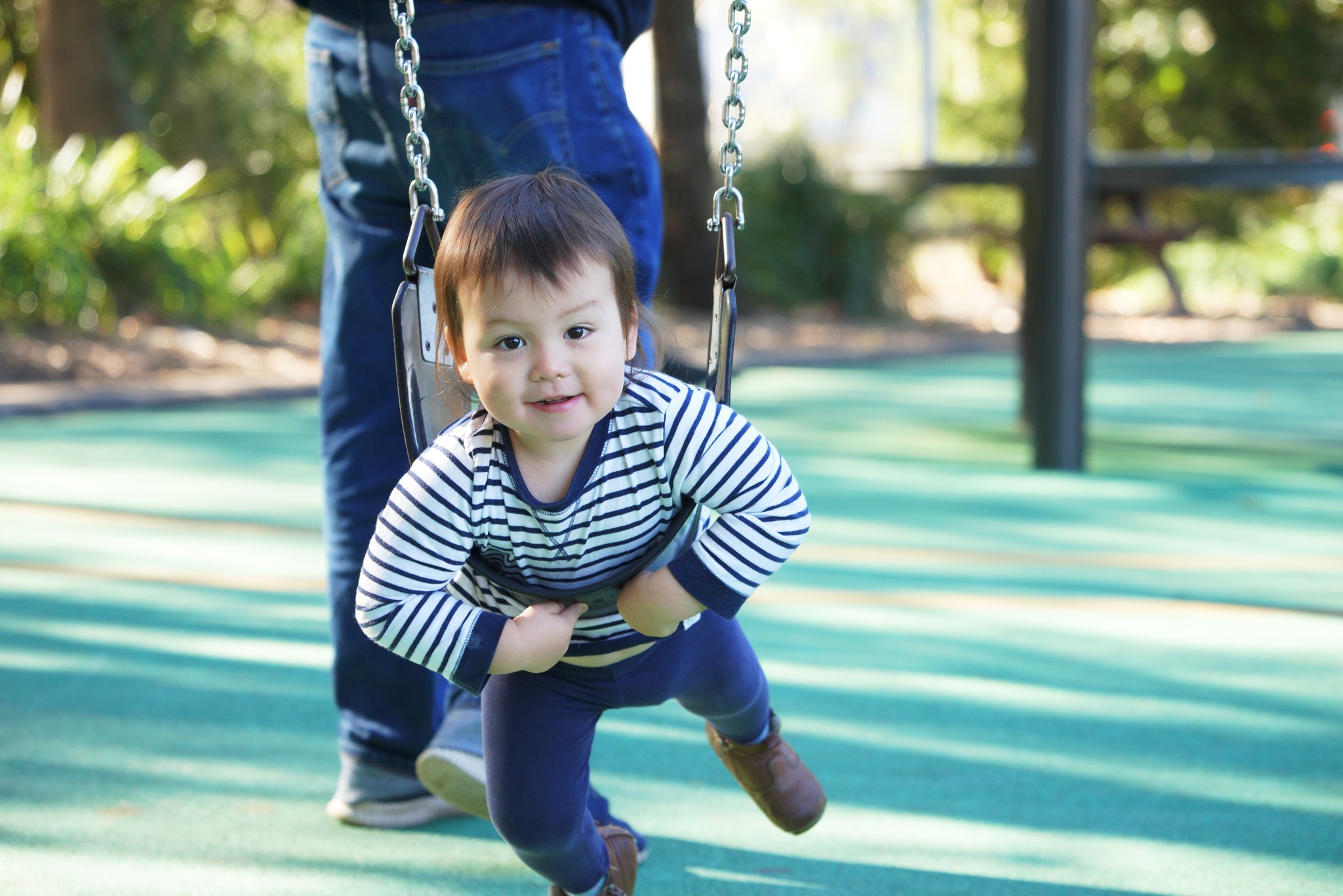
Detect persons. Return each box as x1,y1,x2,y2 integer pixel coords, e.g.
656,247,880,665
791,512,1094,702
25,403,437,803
355,166,828,896
296,0,659,862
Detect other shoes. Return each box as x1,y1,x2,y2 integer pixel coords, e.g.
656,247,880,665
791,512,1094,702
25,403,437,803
328,752,470,829
415,746,646,862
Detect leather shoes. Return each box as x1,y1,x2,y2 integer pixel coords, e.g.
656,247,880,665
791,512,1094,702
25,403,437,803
704,707,828,835
549,819,638,896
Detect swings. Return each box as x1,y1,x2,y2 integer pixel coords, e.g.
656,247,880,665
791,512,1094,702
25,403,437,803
381,0,752,672
